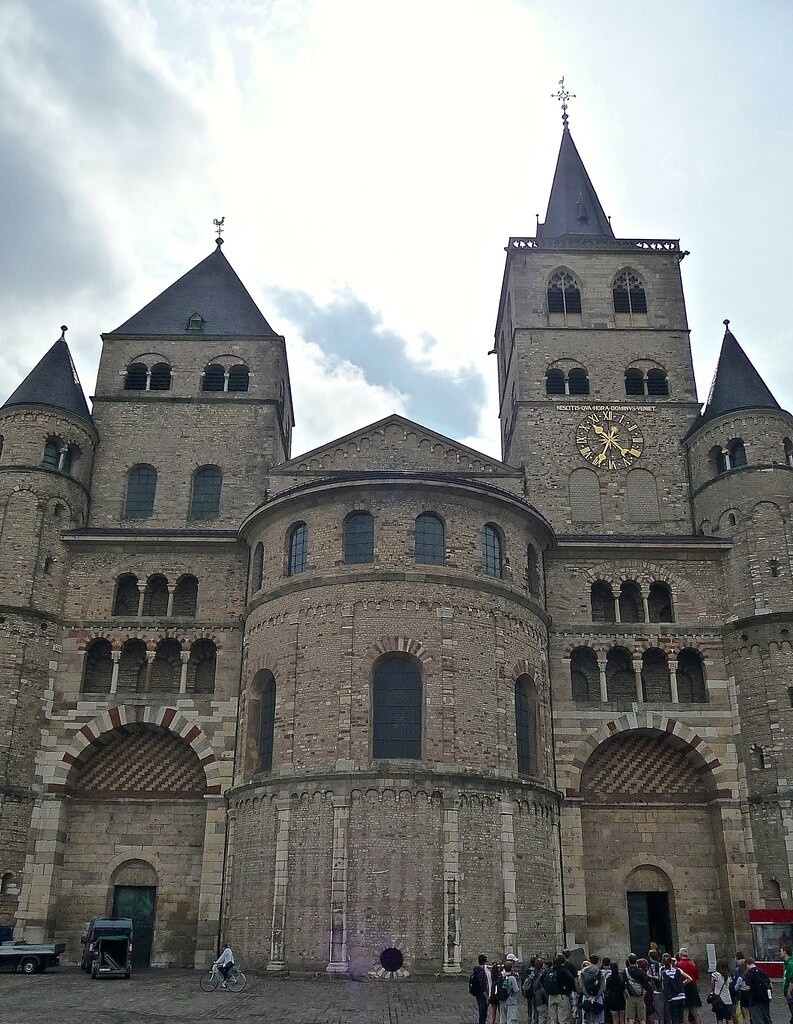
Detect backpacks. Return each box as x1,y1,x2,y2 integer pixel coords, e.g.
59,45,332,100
494,975,513,1000
602,969,612,991
544,968,567,995
469,969,483,996
663,969,683,1000
522,972,542,999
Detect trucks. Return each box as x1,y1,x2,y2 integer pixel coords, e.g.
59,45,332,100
0,923,66,973
89,936,133,980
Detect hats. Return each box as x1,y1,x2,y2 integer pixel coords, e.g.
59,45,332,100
679,948,688,955
506,953,519,962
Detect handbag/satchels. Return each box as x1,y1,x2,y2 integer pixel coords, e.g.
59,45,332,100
707,994,721,1006
626,968,643,995
767,986,773,999
584,971,601,996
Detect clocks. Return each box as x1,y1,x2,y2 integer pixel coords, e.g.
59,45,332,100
575,409,646,472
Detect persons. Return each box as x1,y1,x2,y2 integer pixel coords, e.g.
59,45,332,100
468,940,793,1024
213,943,236,989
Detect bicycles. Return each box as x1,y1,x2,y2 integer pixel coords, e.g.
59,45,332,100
200,959,247,994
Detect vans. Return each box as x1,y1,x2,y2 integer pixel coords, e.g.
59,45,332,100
79,918,134,974
